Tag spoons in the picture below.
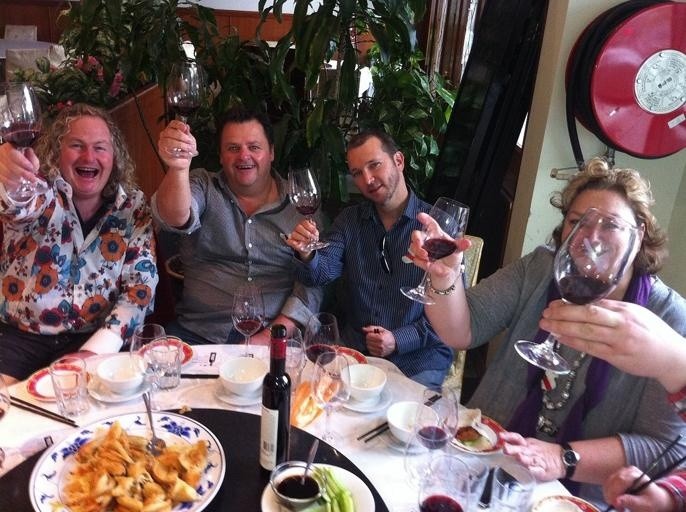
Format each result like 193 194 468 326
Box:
142 392 166 456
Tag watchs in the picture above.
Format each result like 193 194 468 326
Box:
555 439 580 481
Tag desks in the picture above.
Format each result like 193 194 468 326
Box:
0 38 56 61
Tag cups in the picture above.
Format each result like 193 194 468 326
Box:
150 334 181 391
490 464 535 512
449 459 491 511
418 457 470 511
52 355 90 419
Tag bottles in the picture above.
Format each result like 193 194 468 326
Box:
258 324 293 473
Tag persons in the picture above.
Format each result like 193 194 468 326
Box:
0 103 160 392
151 103 329 348
409 155 686 512
287 130 468 393
537 298 685 512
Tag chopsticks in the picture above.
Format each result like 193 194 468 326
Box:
7 394 79 430
605 435 686 512
358 394 442 444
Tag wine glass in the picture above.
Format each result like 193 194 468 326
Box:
165 60 204 156
130 321 165 413
400 198 471 305
313 352 349 440
233 286 265 357
305 313 341 373
415 388 458 470
0 81 47 199
280 326 308 390
515 209 644 374
288 169 330 252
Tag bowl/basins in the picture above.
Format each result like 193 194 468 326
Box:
97 355 146 396
342 364 387 396
387 401 437 442
215 358 267 395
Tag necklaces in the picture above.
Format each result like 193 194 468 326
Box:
539 348 586 416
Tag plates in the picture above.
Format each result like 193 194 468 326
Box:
532 495 603 512
317 347 367 377
140 339 191 367
213 385 263 407
28 411 225 511
29 363 92 403
375 417 449 454
260 465 377 512
86 376 154 403
267 462 323 509
443 417 506 455
340 385 394 412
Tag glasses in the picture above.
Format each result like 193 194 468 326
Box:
379 233 393 276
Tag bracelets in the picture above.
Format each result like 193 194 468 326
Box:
425 263 464 295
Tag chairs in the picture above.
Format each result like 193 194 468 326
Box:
445 235 485 398
5 49 52 82
143 228 184 345
50 45 77 70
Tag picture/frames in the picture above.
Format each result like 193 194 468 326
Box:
4 25 38 42
